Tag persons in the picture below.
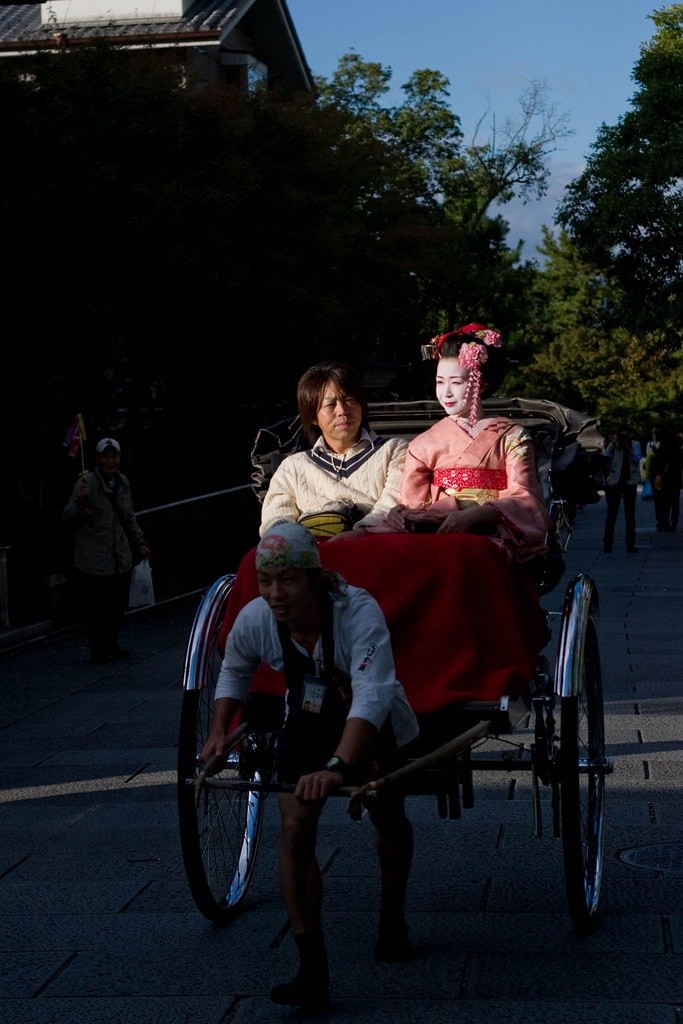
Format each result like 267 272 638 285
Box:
388 323 552 569
303 701 310 711
646 429 683 533
259 362 410 544
602 423 640 553
60 437 150 664
200 523 413 1002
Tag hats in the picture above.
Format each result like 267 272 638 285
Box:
96 438 121 452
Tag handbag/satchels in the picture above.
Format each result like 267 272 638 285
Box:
641 480 653 499
128 558 155 608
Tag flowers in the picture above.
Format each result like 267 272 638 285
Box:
457 342 489 426
475 330 502 348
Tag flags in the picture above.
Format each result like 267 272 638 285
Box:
64 415 86 456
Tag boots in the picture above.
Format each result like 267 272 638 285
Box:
270 932 330 1005
374 877 406 962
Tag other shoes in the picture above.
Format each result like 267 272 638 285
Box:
105 643 141 664
627 546 639 553
74 641 102 665
604 547 612 553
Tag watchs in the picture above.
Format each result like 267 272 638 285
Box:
326 757 349 781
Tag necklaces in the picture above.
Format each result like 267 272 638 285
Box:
330 451 348 480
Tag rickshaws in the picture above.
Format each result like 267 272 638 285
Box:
176 397 613 922
